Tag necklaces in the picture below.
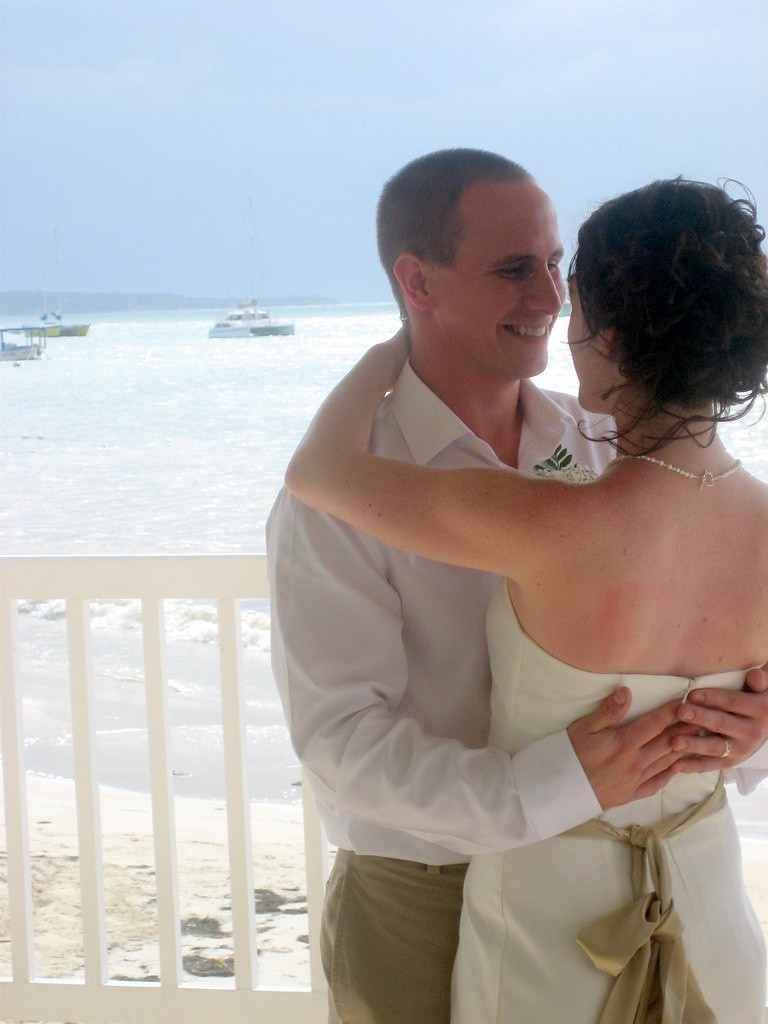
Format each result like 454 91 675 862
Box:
607 455 742 491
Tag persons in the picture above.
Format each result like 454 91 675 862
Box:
260 147 767 1024
277 176 766 1023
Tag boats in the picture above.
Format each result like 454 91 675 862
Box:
0 328 44 361
209 302 294 337
22 322 89 336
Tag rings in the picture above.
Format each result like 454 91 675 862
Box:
720 737 732 758
